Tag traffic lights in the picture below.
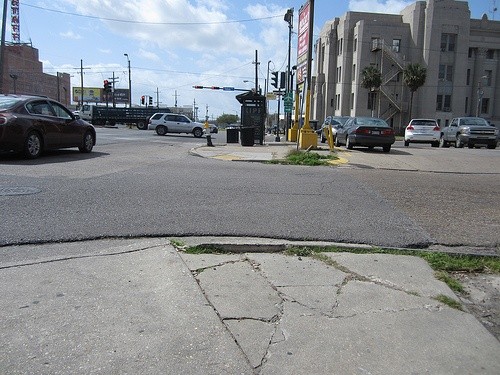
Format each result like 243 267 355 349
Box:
271 72 278 88
149 96 153 105
103 80 109 93
141 96 145 104
108 82 112 92
210 86 220 90
195 85 204 90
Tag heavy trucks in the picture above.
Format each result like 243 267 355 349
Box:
68 104 172 130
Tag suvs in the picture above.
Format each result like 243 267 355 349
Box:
147 113 205 138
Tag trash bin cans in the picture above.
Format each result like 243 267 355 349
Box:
226 129 239 143
240 127 254 147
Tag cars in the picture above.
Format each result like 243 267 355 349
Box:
267 125 285 135
0 93 97 160
334 116 395 153
319 116 353 145
402 119 441 148
442 117 497 149
208 124 218 135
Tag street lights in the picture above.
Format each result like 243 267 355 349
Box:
9 73 18 93
124 53 132 108
476 76 489 118
